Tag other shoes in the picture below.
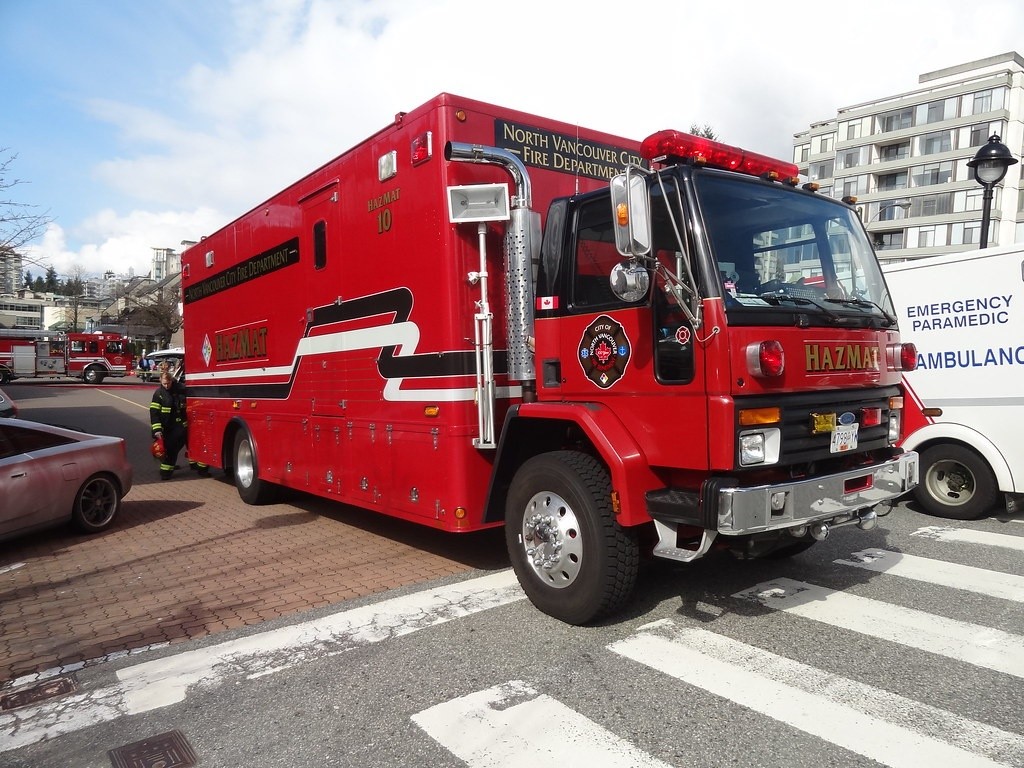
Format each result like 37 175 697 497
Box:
195 466 211 478
162 473 171 480
175 465 181 470
190 464 198 469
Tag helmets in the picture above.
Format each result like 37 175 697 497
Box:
151 436 166 459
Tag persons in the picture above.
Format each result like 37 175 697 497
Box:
149 371 214 482
174 361 182 373
156 356 171 381
138 351 153 383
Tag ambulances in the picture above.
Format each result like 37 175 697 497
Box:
797 241 1024 521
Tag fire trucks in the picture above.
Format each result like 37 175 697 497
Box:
181 91 917 631
0 328 134 386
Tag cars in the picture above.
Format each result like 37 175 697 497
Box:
0 388 18 419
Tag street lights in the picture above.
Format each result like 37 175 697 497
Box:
966 130 1019 249
850 200 911 297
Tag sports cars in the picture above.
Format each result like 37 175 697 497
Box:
0 417 134 548
